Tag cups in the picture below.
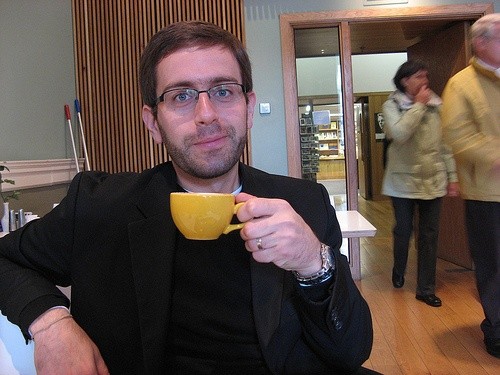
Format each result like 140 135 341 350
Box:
170 192 252 241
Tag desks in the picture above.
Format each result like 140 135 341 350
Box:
317 159 346 180
335 210 377 281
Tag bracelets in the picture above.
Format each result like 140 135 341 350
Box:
33 313 74 340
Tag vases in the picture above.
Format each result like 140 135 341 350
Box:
0 203 10 233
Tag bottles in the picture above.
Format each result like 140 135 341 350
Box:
18 209 25 228
10 210 16 231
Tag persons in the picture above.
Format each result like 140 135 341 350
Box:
440 11 500 357
0 16 391 375
383 58 459 307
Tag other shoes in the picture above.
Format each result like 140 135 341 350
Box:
415 293 442 307
392 268 404 288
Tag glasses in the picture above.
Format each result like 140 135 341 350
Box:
154 82 249 112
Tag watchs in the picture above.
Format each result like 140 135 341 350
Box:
293 241 335 285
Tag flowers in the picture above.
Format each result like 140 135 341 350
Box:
0 161 21 203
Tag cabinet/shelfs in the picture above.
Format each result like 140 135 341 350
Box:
300 114 319 182
318 120 342 155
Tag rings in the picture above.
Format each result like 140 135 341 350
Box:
255 237 264 251
